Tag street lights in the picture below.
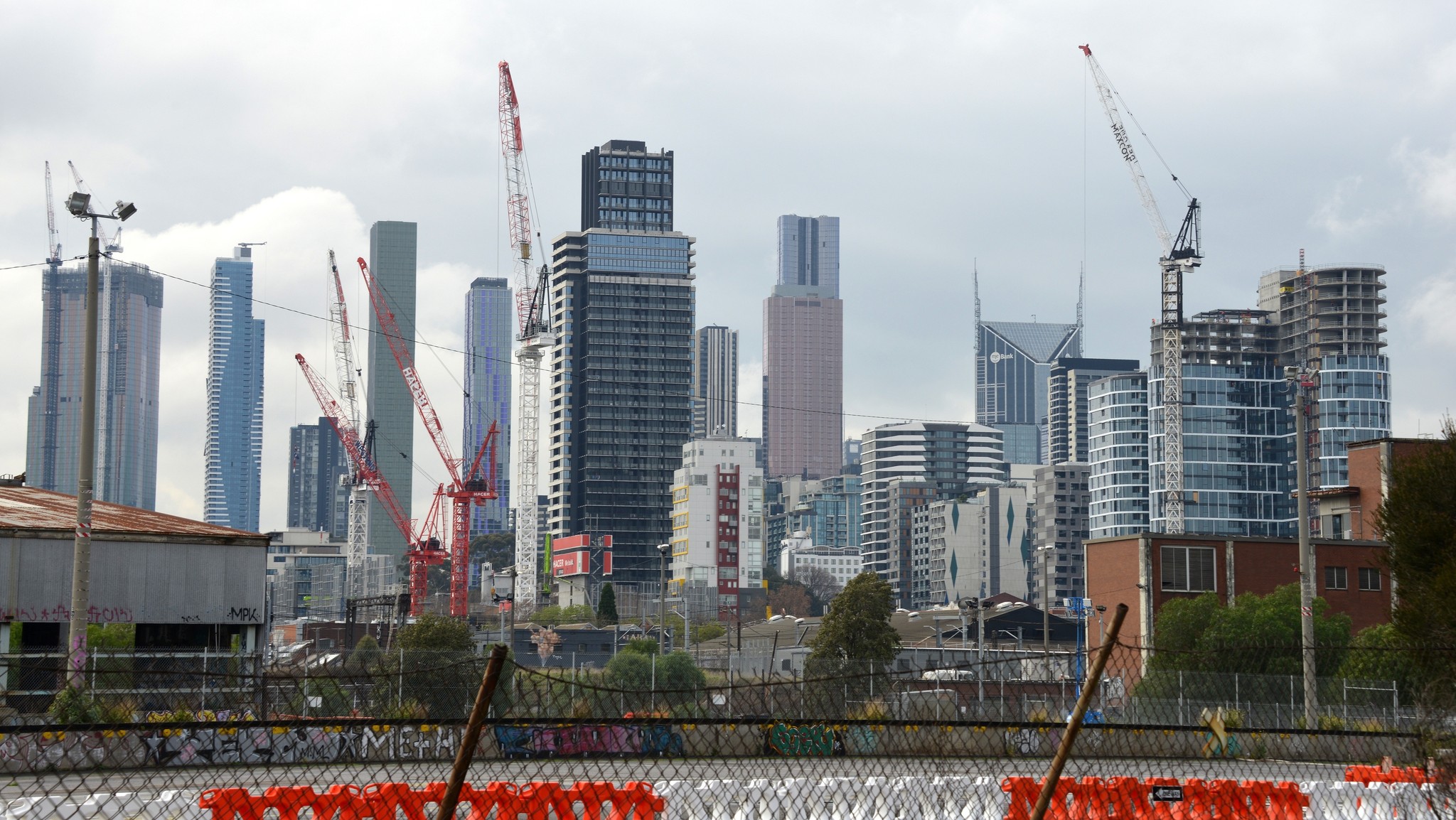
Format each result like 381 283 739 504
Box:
61 188 138 695
658 544 670 654
1038 545 1054 679
1096 605 1106 712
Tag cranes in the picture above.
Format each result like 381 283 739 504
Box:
358 257 501 616
295 352 449 618
67 159 124 501
40 160 64 491
494 57 558 622
326 249 382 618
1076 43 1207 534
